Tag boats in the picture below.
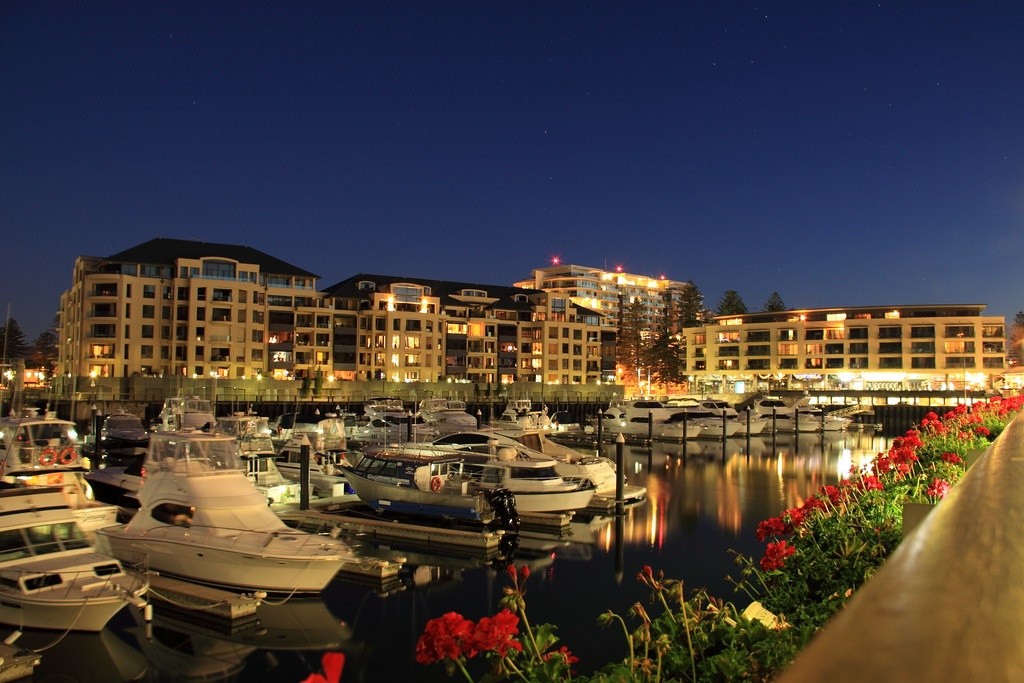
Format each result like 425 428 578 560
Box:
650 440 702 458
0 628 44 683
566 511 619 546
359 536 508 589
0 397 855 633
823 429 851 442
734 435 766 453
514 526 570 576
136 562 353 683
0 620 150 683
768 432 819 446
698 438 740 457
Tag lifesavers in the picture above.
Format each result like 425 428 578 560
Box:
39 449 57 466
59 447 78 464
431 477 441 492
